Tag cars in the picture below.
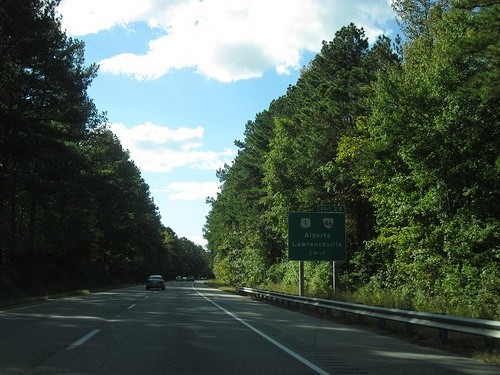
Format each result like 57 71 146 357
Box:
175 275 195 282
146 275 165 290
